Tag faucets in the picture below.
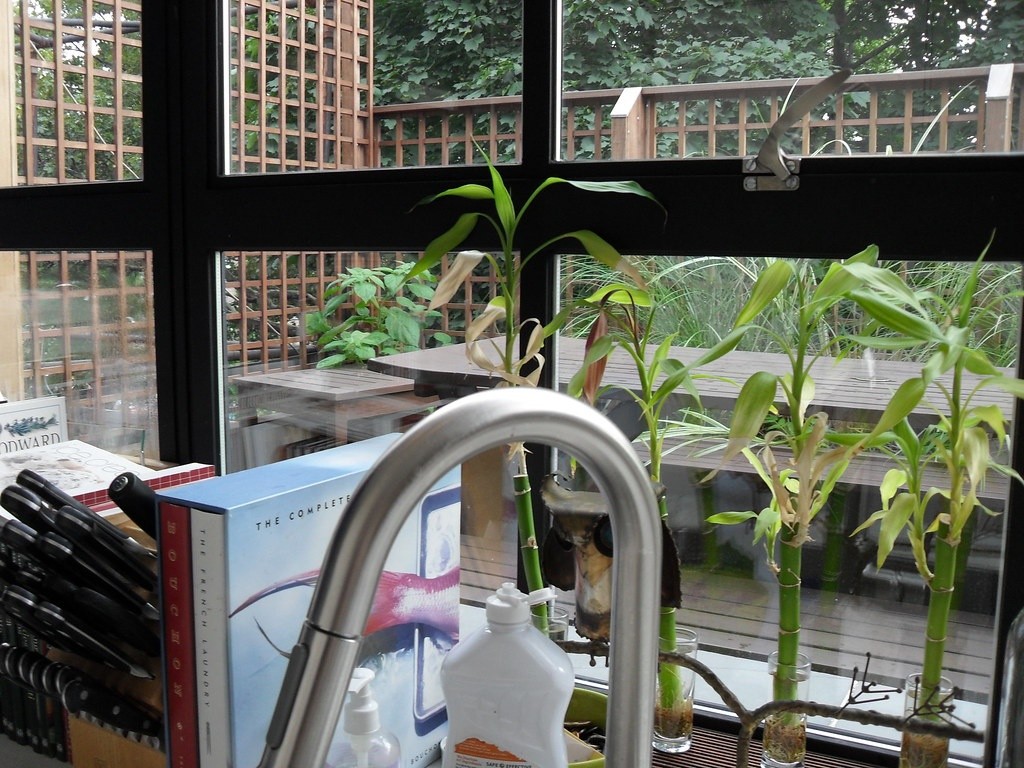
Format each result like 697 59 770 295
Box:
258 386 661 768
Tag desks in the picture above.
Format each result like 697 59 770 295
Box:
370 332 1018 565
230 369 438 448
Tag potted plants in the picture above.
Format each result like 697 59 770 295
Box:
714 243 930 768
549 252 739 753
840 228 1019 768
396 135 659 639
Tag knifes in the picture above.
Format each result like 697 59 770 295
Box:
0 469 159 750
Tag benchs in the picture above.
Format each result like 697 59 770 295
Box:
630 438 1010 557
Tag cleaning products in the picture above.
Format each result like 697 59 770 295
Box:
324 667 401 767
440 581 576 768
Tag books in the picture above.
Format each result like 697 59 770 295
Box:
0 395 215 526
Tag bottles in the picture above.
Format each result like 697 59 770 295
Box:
440 583 575 768
326 667 402 768
